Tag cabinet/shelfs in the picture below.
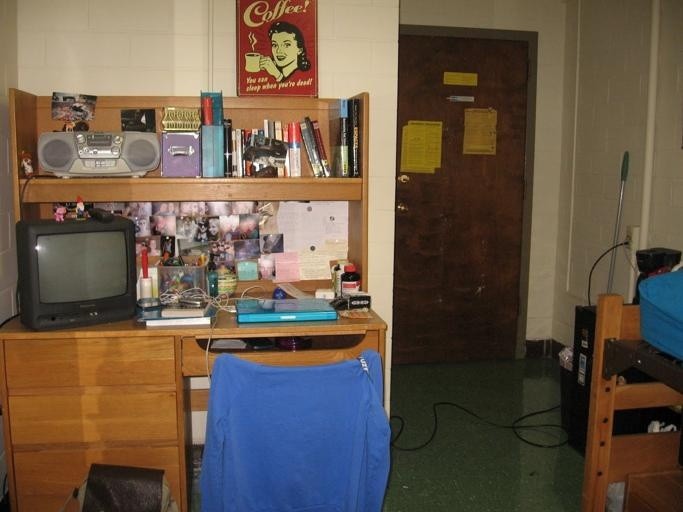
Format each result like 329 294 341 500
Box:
181 292 385 512
9 88 370 211
3 311 186 509
581 294 682 510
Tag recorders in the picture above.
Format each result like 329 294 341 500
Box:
38 130 161 180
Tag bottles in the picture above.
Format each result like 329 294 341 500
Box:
340 265 360 297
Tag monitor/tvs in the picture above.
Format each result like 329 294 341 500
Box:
15 214 138 331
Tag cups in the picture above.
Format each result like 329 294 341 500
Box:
244 53 264 73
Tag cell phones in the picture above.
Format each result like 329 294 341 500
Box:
179 297 205 308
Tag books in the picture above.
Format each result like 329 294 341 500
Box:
223 98 359 180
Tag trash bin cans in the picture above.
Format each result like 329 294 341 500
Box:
559 347 575 432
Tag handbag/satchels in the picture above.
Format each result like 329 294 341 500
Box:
75 462 180 511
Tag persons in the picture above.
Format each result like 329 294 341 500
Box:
128 201 282 267
